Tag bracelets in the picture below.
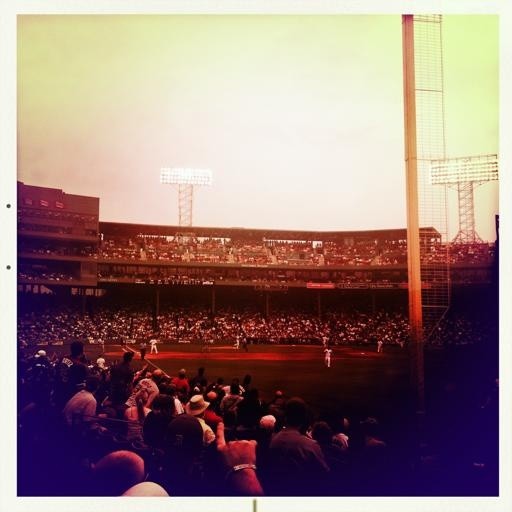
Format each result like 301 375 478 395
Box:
225 464 256 480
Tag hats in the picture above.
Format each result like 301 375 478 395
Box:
260 415 276 428
186 394 209 416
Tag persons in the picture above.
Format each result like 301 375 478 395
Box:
98 337 105 352
245 415 276 448
265 311 322 345
383 340 409 350
95 352 140 496
241 335 248 352
235 311 265 335
234 334 240 350
19 271 77 280
218 374 269 421
217 422 264 497
86 305 155 338
215 311 234 344
17 309 86 345
322 311 377 345
378 310 409 339
150 337 158 354
324 346 332 367
140 340 147 361
100 230 276 283
156 306 215 345
121 338 123 347
23 243 100 258
269 397 330 497
311 411 386 467
424 311 481 345
17 339 98 497
248 335 265 344
377 339 383 352
273 240 496 282
141 364 226 496
